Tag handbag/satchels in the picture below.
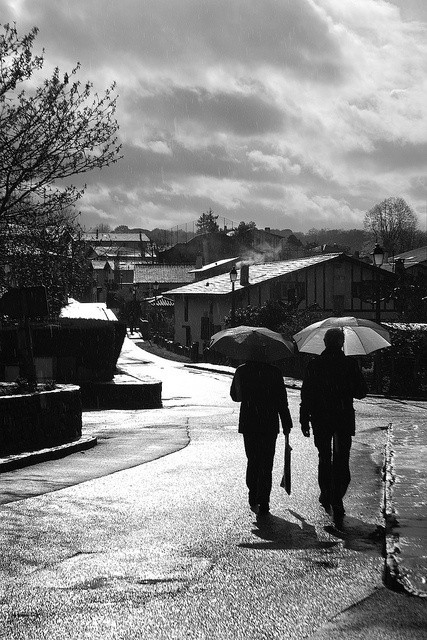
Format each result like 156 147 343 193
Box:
280 434 293 495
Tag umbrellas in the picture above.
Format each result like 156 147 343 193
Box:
293 316 392 356
209 326 293 363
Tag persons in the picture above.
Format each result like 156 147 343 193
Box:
230 349 293 515
119 312 127 336
128 312 135 335
300 329 367 520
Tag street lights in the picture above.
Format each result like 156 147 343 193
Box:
368 243 383 323
230 266 236 327
119 273 123 290
133 283 138 331
153 281 159 343
